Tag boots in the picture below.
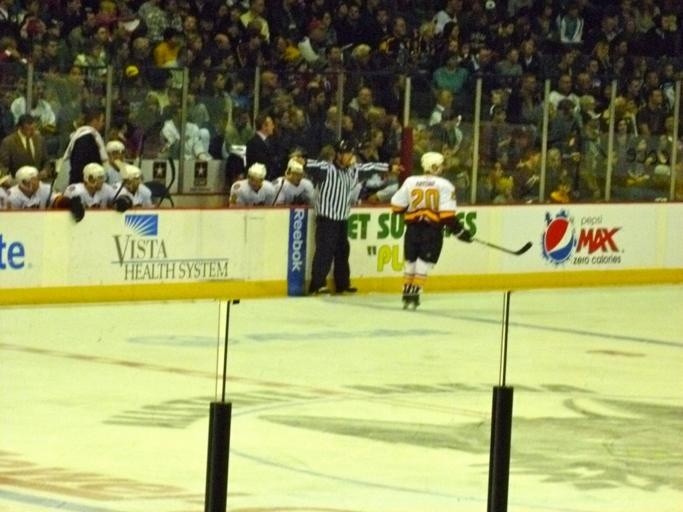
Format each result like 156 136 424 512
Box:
402 282 420 305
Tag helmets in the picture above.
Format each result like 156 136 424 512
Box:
120 164 142 181
248 163 267 181
107 140 126 162
285 160 304 186
15 165 39 191
421 152 445 175
332 139 358 154
83 163 107 189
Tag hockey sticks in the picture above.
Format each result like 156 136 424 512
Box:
155 156 175 208
109 160 130 210
473 237 532 256
45 160 57 210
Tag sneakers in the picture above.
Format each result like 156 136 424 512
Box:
312 286 331 293
335 287 357 293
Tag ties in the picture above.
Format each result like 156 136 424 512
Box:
25 137 33 158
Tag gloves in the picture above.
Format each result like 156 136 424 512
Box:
451 223 473 243
68 195 85 222
114 194 132 213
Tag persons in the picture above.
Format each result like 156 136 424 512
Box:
393 150 474 310
0 0 682 224
292 136 407 298
